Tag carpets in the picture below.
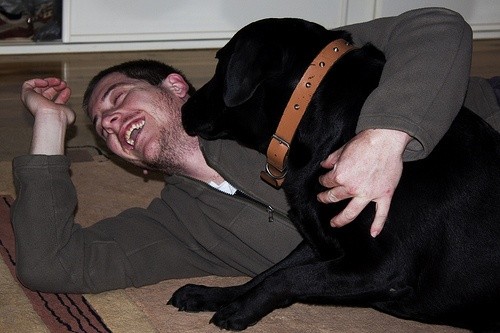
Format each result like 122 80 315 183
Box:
0 146 481 333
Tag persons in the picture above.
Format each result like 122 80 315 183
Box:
11 6 500 293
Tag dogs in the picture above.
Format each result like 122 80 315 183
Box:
165 17 500 333
